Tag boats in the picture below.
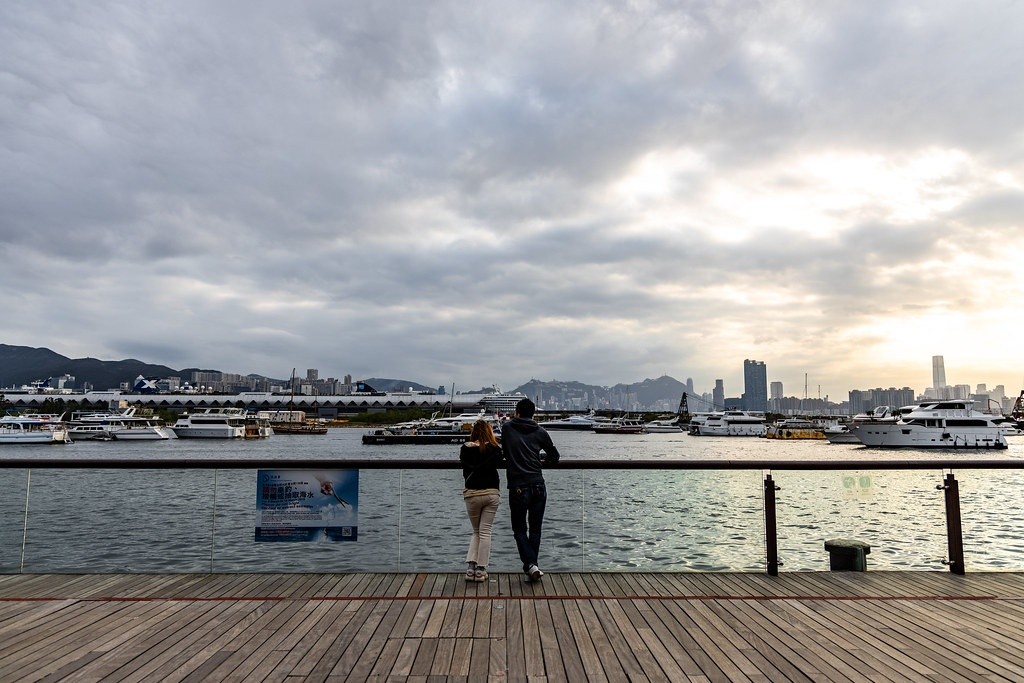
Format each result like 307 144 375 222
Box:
814 399 1024 450
536 408 768 436
383 382 511 434
0 368 328 444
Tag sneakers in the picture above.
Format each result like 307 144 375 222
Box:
475 569 489 581
465 569 475 582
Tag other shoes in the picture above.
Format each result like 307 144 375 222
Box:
524 574 531 582
529 564 544 580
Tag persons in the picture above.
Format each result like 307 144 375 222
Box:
502 398 560 582
459 419 503 582
